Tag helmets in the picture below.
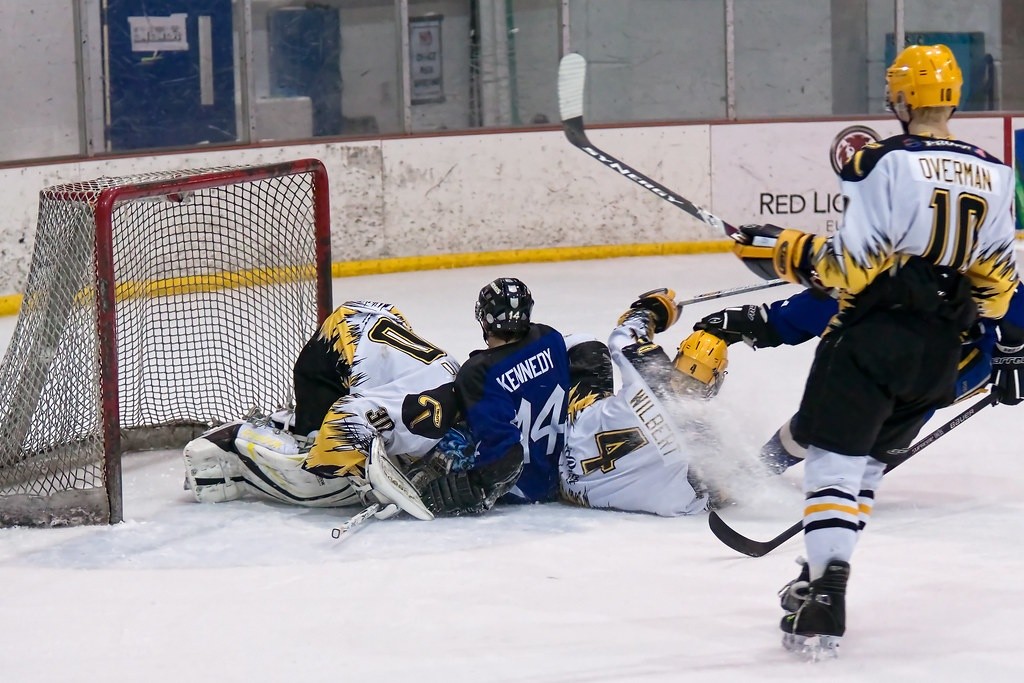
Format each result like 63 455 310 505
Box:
885 45 963 112
474 278 535 333
674 330 729 386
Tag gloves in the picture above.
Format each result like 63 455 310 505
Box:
734 224 811 283
691 302 769 350
422 471 483 518
631 287 684 332
990 326 1024 406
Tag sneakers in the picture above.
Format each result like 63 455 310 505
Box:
778 562 810 611
780 560 850 637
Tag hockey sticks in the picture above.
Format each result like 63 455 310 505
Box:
705 391 995 559
326 497 387 542
555 50 747 244
674 280 794 308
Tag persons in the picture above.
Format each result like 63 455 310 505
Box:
734 43 1020 663
183 279 1024 518
